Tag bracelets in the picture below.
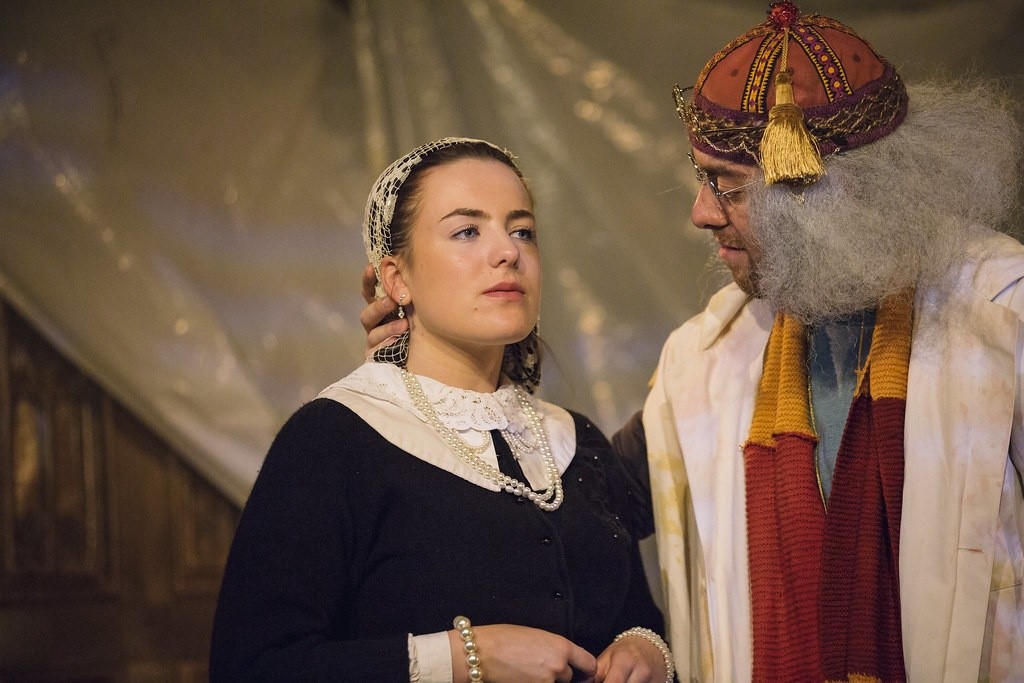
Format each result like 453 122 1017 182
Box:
453 615 483 682
613 626 676 683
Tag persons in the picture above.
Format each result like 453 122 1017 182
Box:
206 137 681 683
358 0 1024 683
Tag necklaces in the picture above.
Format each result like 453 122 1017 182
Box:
401 364 565 512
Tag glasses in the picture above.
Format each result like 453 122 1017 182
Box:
686 151 771 213
671 83 770 144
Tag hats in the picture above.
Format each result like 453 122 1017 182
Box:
687 0 910 186
